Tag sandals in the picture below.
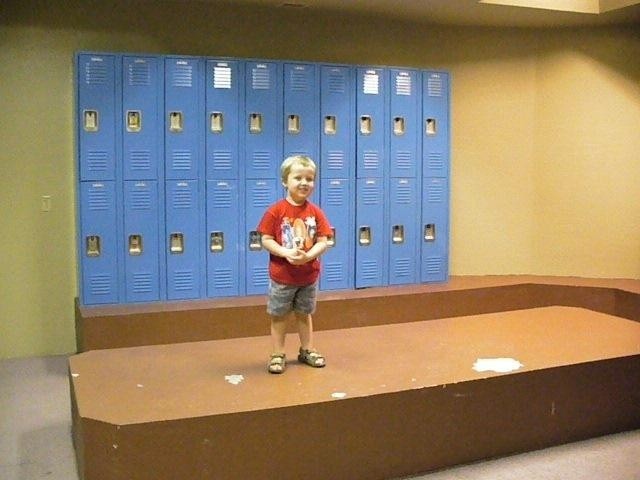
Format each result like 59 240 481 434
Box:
299 347 325 367
268 352 285 373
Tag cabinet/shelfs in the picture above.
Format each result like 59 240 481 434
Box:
75 51 450 307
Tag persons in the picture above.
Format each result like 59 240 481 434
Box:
257 154 335 374
